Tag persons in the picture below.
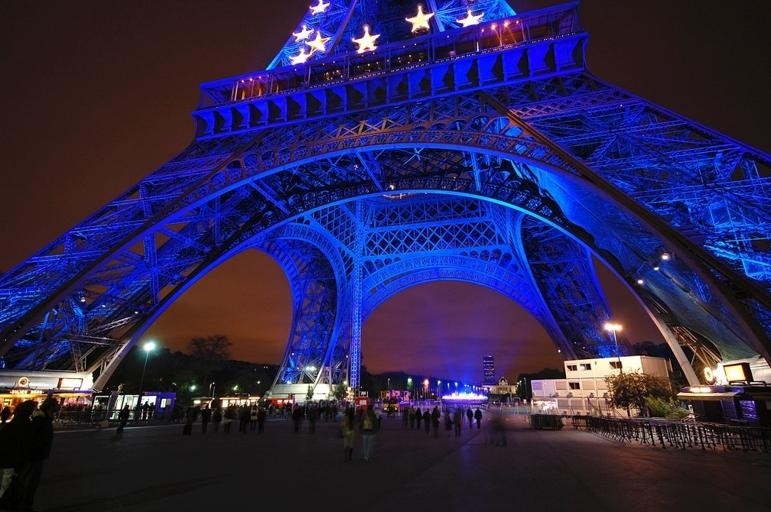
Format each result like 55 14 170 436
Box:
116 405 130 433
172 402 379 462
133 403 155 424
1 401 38 512
31 395 59 460
403 403 483 438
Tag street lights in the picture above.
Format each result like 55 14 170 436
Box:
515 376 529 401
385 377 473 400
207 382 216 396
603 320 635 419
134 341 156 410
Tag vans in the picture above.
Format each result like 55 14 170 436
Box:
383 396 399 412
